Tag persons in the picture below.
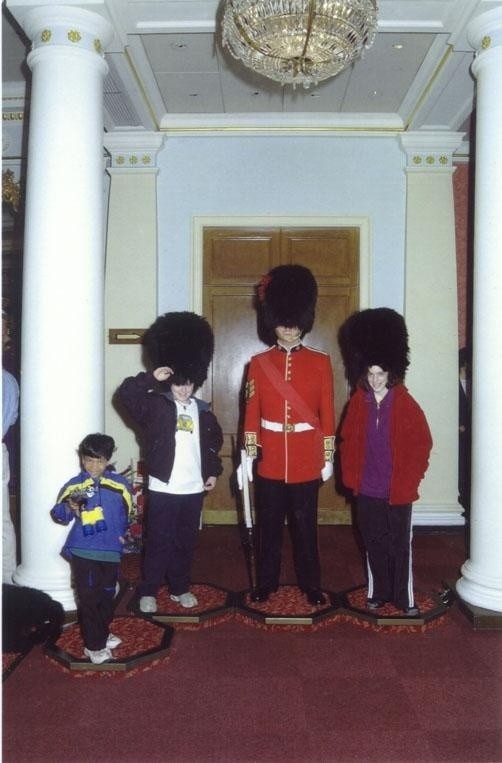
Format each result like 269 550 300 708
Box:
240 266 334 607
2 307 20 584
112 311 225 613
337 308 433 613
50 432 133 664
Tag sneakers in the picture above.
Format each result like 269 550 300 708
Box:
140 595 159 614
84 646 113 665
170 591 199 608
105 633 123 650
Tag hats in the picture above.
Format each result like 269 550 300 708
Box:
255 264 318 347
143 312 214 391
338 308 409 388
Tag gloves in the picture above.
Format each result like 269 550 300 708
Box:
236 458 254 488
321 461 335 481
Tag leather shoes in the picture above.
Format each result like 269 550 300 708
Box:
251 584 279 603
396 600 420 618
366 597 389 610
303 587 327 605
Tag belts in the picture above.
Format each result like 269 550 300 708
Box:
261 418 315 433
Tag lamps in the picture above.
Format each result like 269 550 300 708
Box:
216 0 379 93
